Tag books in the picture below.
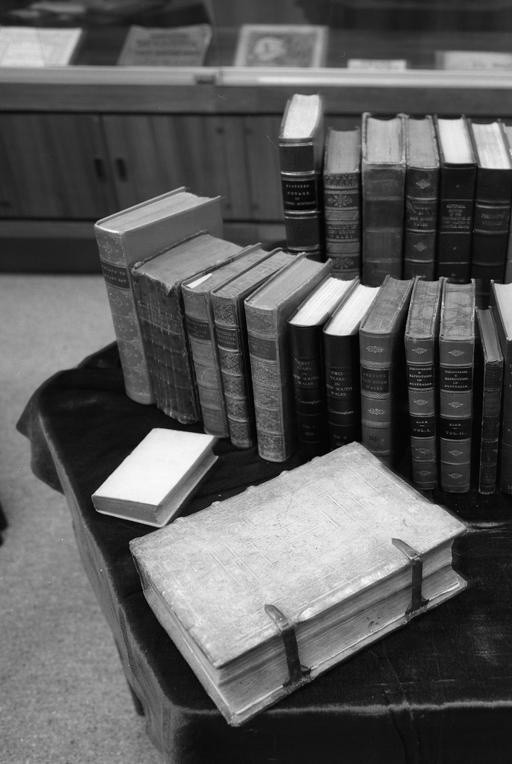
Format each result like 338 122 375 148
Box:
275 93 512 308
347 58 406 74
131 230 511 540
128 440 471 728
1 27 84 66
118 25 212 65
95 185 221 404
92 428 219 528
434 50 512 73
234 23 329 68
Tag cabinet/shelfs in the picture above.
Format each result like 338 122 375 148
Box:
1 68 510 277
17 340 510 699
